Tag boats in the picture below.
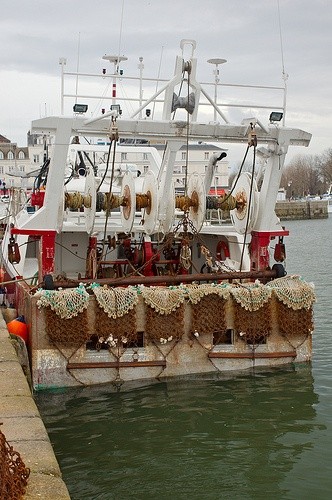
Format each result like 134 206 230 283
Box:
2 35 316 386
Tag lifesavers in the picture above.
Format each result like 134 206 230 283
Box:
216 241 229 261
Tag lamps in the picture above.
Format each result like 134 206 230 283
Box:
269 112 283 121
73 103 88 112
110 105 121 112
